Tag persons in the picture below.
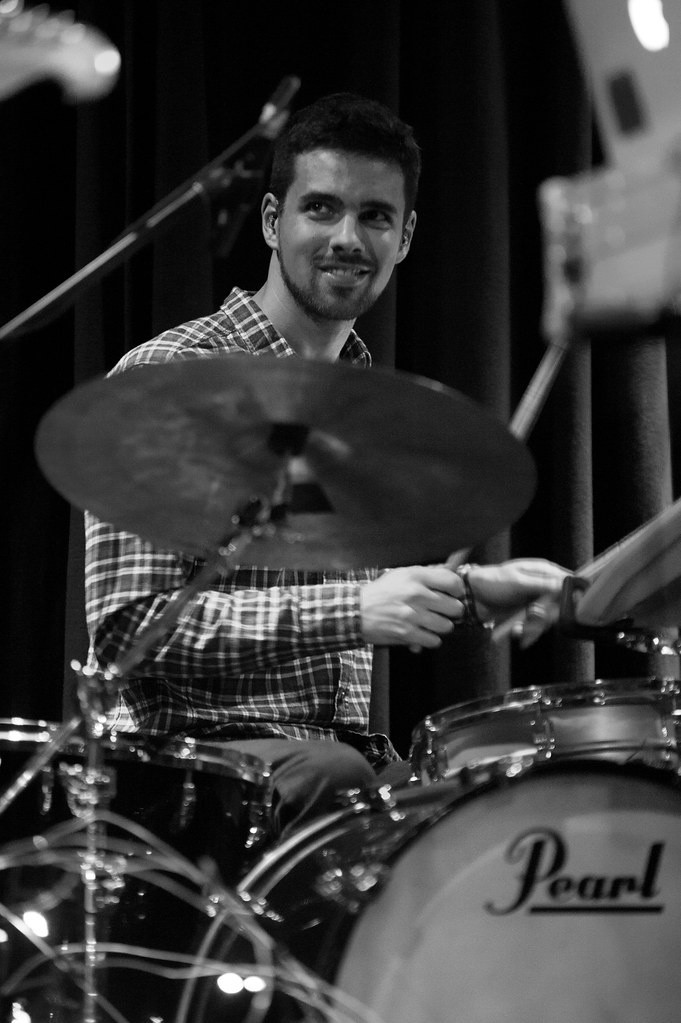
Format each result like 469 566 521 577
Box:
84 94 577 848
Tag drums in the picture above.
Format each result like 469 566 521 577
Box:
0 719 281 1022
179 761 681 1023
412 668 680 773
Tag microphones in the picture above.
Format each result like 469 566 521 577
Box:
235 75 299 180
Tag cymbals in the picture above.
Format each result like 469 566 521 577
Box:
30 351 541 582
